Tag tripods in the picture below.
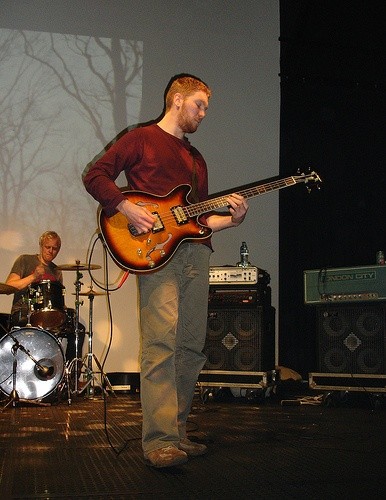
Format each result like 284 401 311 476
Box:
0 325 52 412
59 267 120 403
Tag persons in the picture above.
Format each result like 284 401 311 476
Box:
83 77 250 468
6 231 85 385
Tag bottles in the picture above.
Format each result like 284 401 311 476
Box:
240 242 249 268
376 249 385 266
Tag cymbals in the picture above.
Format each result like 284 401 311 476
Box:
53 261 103 272
0 283 20 295
72 289 111 297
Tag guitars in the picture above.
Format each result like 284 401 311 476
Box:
97 164 324 275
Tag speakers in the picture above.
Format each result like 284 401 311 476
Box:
197 303 278 390
310 303 386 394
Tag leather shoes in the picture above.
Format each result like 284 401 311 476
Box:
179 439 208 456
147 444 188 469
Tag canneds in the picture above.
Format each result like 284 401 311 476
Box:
376 251 384 265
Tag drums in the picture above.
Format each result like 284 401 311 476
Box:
47 307 76 337
0 327 66 401
22 279 67 329
